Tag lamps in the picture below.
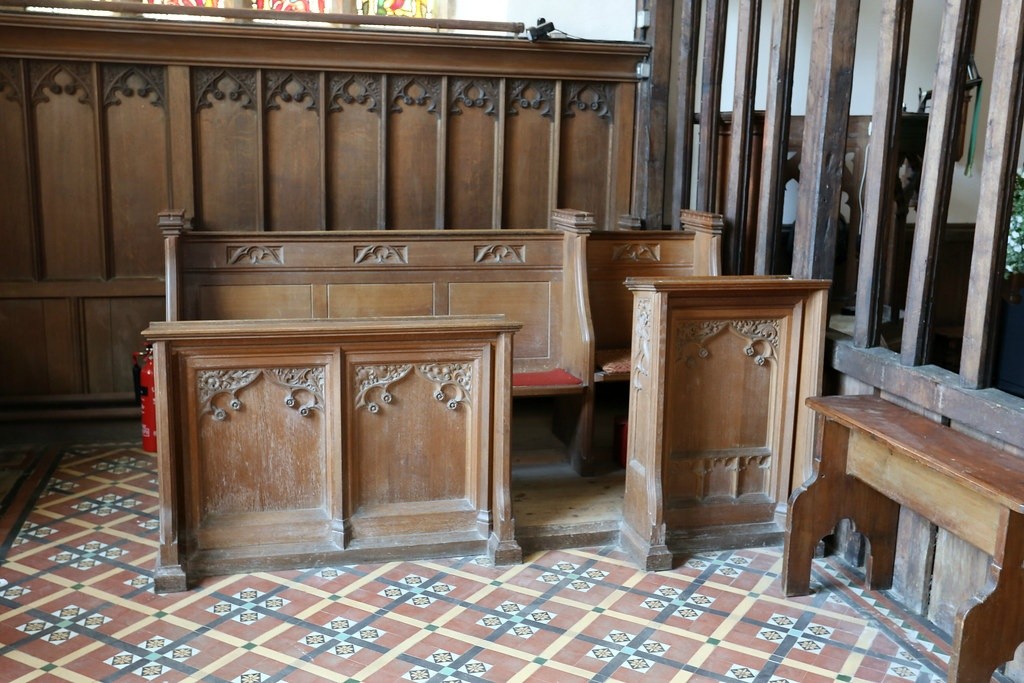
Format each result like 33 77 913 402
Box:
526 18 555 43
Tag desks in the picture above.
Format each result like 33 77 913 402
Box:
782 393 1024 683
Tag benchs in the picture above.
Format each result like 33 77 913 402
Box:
157 208 724 478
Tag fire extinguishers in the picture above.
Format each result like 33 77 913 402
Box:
132 342 157 452
